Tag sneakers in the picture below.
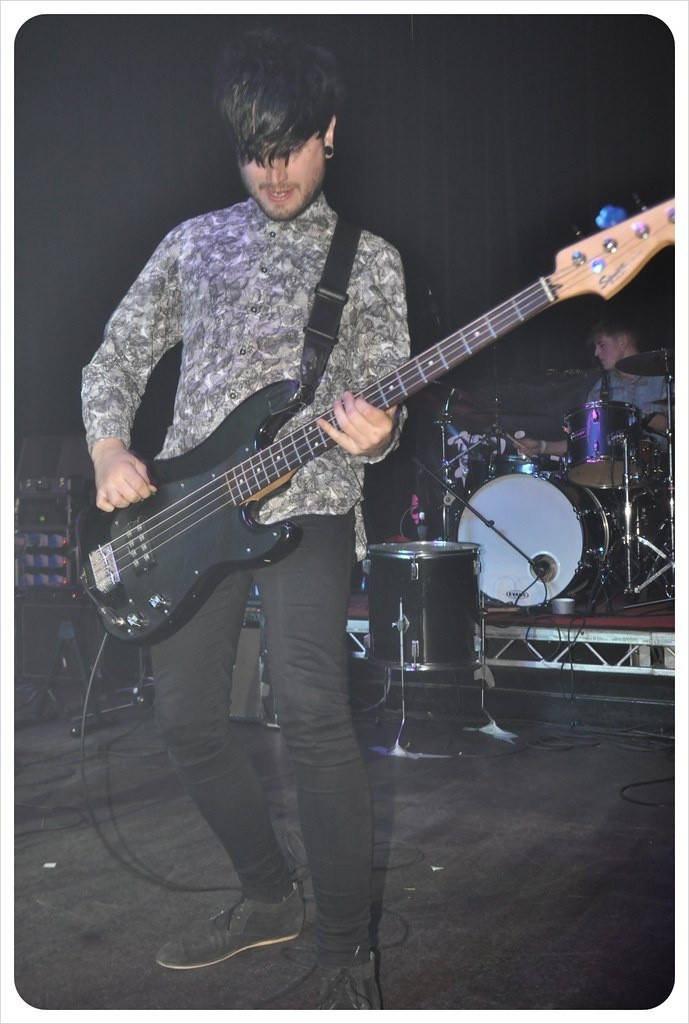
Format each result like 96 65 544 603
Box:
256 946 381 1009
155 881 305 970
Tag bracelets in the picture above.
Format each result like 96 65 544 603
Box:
540 439 546 456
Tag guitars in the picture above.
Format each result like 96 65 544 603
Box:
75 190 675 641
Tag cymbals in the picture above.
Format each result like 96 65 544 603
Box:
615 348 675 376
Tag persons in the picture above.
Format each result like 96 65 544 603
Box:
81 42 410 1010
513 312 675 457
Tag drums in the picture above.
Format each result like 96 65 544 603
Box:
365 400 645 672
